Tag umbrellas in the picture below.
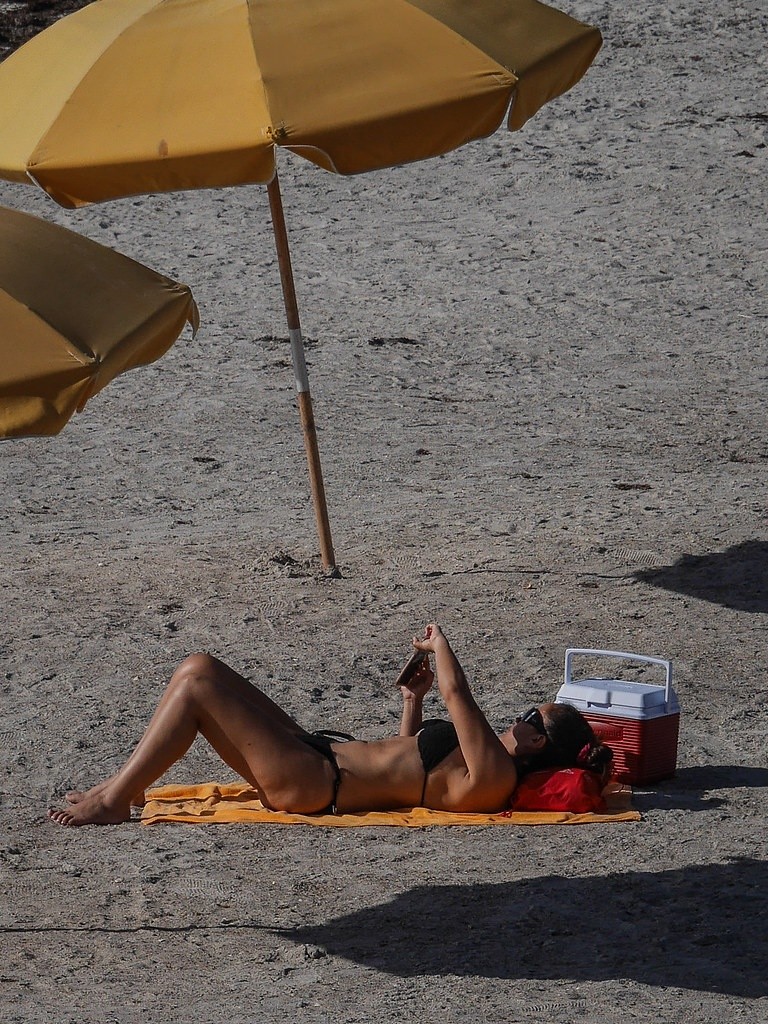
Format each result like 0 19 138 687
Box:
0 0 602 570
0 207 200 442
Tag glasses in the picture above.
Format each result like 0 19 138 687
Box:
520 707 547 736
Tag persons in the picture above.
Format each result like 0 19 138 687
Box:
46 623 614 826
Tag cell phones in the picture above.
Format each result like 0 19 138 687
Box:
394 637 432 687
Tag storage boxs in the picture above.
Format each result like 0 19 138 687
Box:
554 645 682 788
